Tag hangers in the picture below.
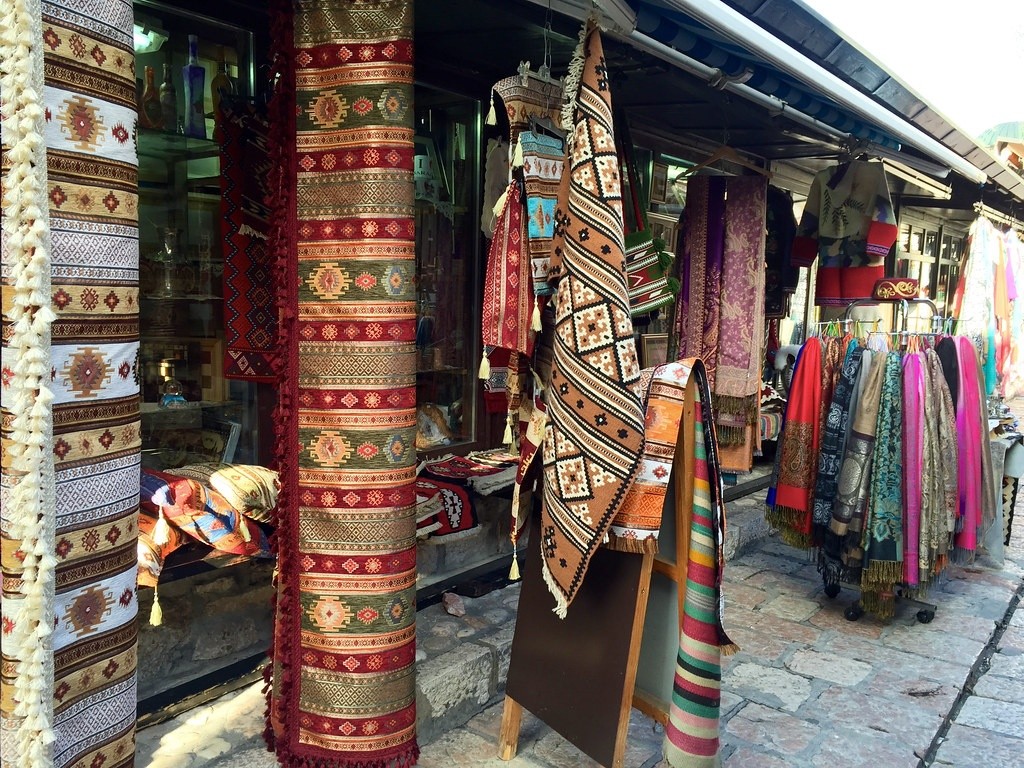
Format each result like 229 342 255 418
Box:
518 22 564 90
216 64 272 113
674 129 774 185
527 82 568 153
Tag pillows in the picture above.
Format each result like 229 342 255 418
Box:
760 378 787 413
163 462 280 523
140 466 274 558
137 513 196 626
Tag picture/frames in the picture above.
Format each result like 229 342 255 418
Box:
640 334 669 368
148 413 242 464
415 129 451 201
650 162 668 204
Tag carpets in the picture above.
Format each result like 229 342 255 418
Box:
418 8 769 768
216 98 277 384
0 2 141 768
261 0 420 768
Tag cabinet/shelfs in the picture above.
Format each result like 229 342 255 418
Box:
138 127 242 412
414 203 470 463
